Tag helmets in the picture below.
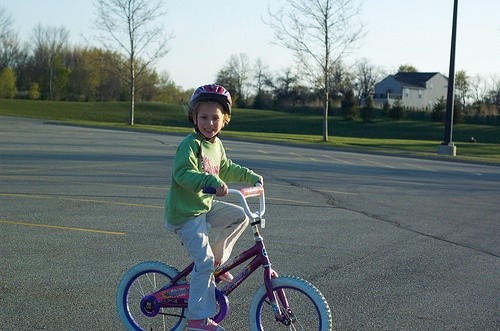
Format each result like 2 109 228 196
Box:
188 84 233 126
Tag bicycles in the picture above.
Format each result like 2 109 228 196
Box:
115 182 333 331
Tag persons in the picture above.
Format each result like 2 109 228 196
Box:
164 84 263 331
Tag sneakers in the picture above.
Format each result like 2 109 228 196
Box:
213 261 233 282
187 318 226 331
